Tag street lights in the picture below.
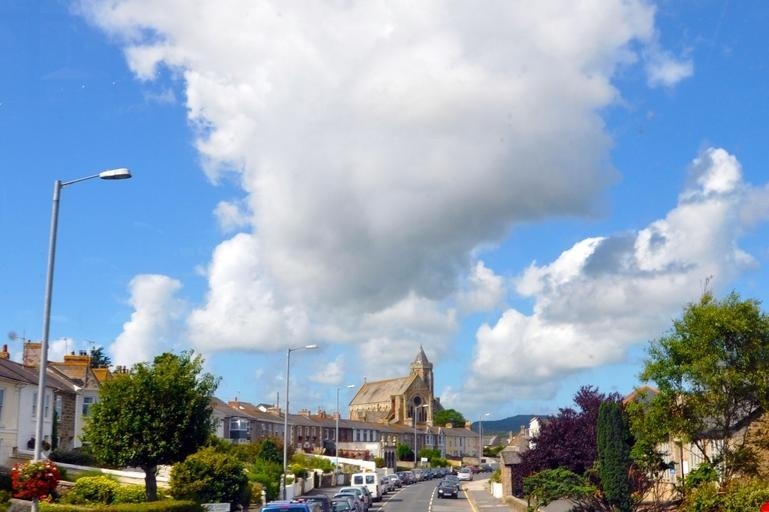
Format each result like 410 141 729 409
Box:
673 392 687 500
283 343 319 501
414 404 429 469
335 385 355 473
33 167 131 462
478 413 490 462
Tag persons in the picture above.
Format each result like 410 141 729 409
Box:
40 434 51 458
28 437 35 450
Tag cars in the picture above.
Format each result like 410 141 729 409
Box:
260 463 492 512
